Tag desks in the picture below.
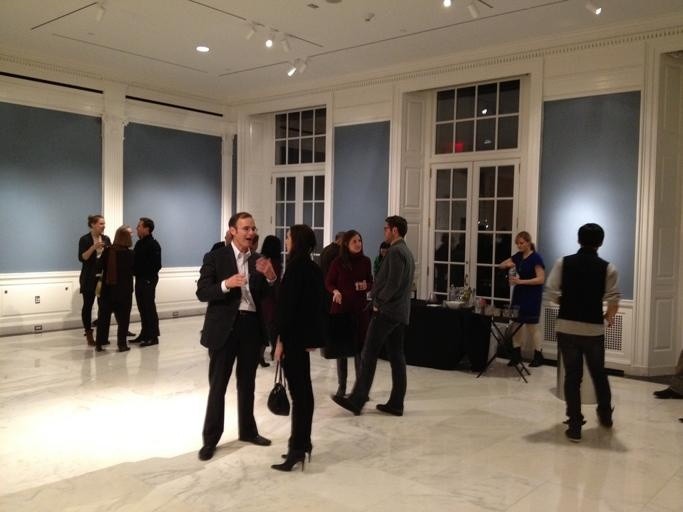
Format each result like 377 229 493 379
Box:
472 308 540 383
368 296 493 375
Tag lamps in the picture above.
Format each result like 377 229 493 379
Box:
286 58 307 77
245 24 291 54
443 1 481 21
95 4 107 23
585 1 603 16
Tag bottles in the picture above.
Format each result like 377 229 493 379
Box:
409 282 417 299
508 266 517 286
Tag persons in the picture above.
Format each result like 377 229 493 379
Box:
652 350 681 402
74 215 136 347
497 231 545 367
212 228 392 397
429 225 509 302
195 213 271 460
544 223 620 443
127 217 163 347
331 216 415 416
95 224 135 352
268 224 319 471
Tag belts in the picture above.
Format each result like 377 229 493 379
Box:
237 310 248 316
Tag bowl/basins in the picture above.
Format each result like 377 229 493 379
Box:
445 300 464 310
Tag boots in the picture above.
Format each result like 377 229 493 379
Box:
529 348 545 367
85 329 95 345
507 346 522 366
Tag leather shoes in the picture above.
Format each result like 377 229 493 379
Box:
200 446 214 459
96 330 158 351
260 357 270 366
239 435 271 445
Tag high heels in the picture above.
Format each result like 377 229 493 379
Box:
272 443 313 471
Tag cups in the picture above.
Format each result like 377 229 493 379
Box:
474 298 521 319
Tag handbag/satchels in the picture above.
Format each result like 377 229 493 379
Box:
268 383 289 415
95 281 102 297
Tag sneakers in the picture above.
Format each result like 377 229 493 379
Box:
565 429 582 442
334 390 369 412
654 388 683 399
377 404 403 416
598 416 613 427
